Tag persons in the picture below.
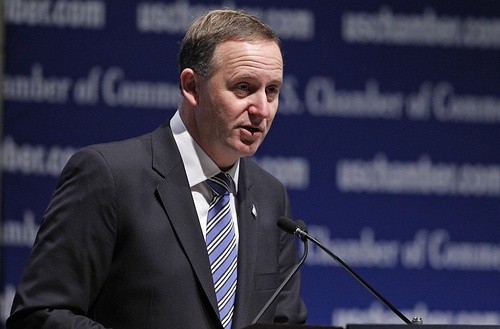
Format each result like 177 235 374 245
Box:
2 7 308 329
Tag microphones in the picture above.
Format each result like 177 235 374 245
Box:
276 216 414 325
251 220 309 325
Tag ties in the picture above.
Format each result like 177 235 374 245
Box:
207 172 239 329
